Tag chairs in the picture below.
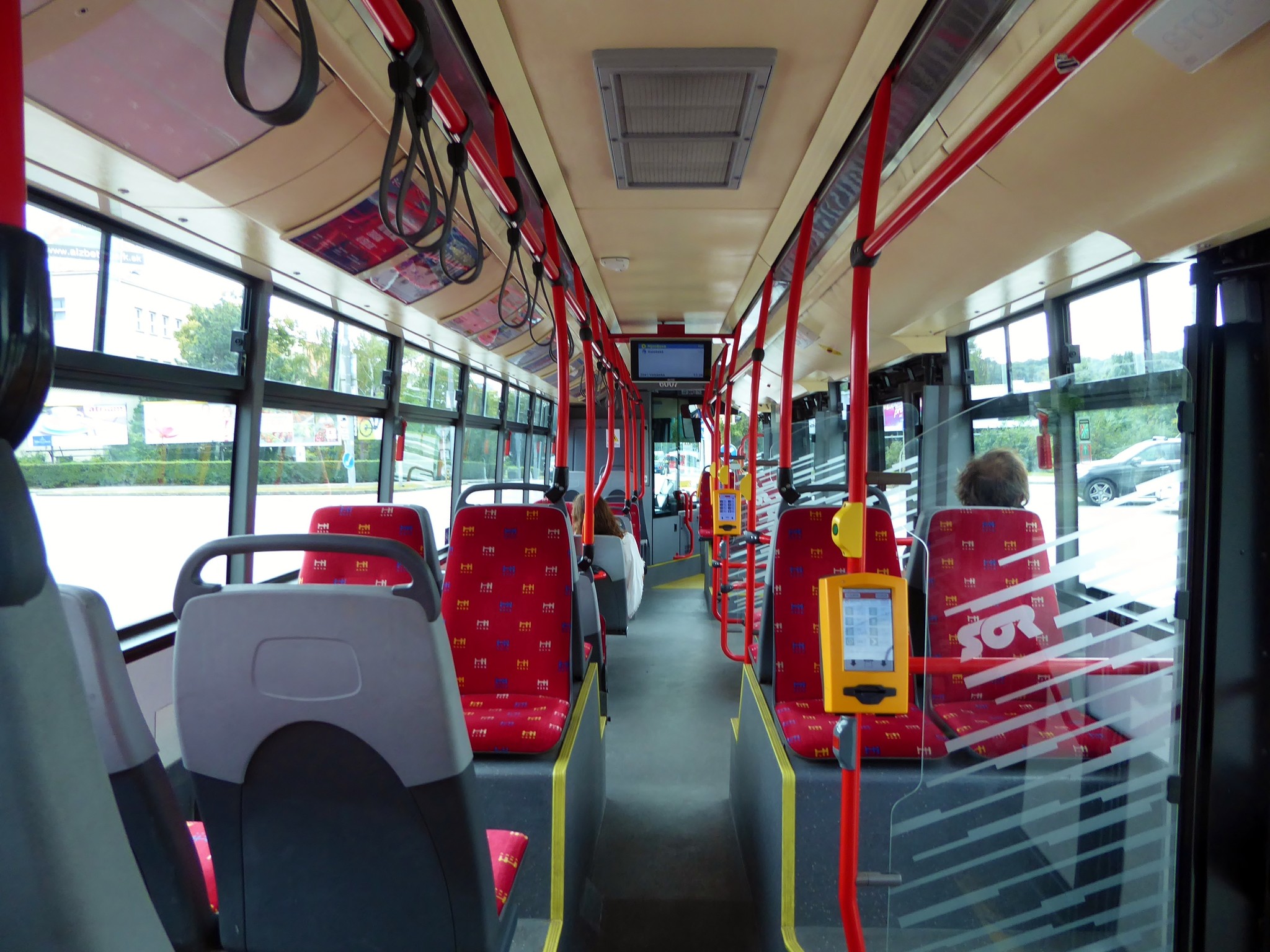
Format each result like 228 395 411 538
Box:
0 465 1131 952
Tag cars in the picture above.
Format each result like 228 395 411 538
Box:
655 462 664 475
667 456 678 468
1076 436 1182 506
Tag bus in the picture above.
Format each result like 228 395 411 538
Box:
680 454 685 474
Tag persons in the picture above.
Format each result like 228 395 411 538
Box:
568 494 647 620
959 449 1033 507
